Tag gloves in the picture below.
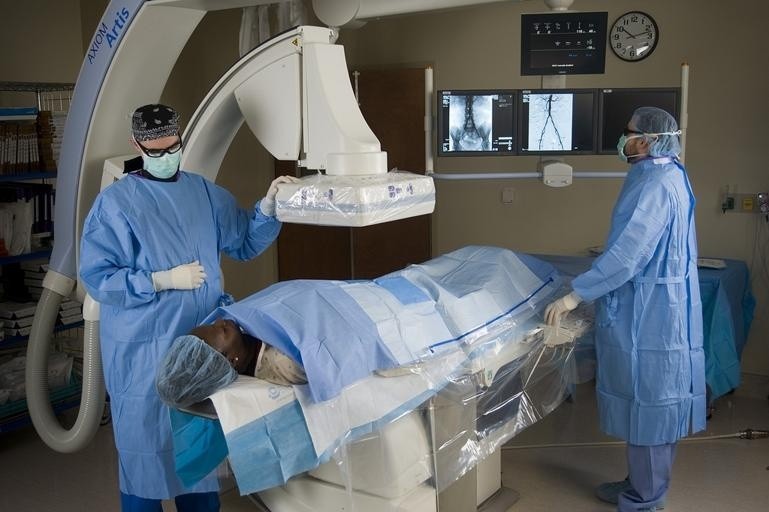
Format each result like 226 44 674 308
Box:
543 289 584 327
150 259 208 295
259 174 301 219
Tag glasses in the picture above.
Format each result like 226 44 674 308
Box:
132 133 183 159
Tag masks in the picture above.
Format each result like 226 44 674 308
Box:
616 133 648 163
133 135 183 179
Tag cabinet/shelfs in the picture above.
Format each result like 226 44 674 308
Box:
0 81 112 427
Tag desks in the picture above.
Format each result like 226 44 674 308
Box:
529 254 755 419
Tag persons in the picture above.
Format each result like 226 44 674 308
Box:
77 104 300 512
158 318 305 411
542 105 696 511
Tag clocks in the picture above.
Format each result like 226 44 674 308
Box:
609 11 659 63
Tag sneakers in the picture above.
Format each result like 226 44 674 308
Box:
595 480 630 505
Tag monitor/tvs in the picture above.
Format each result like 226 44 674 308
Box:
598 87 681 155
436 90 520 158
519 10 608 78
517 87 598 156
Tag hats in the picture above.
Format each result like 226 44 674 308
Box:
131 103 182 140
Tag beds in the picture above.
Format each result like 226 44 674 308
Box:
177 284 595 512
